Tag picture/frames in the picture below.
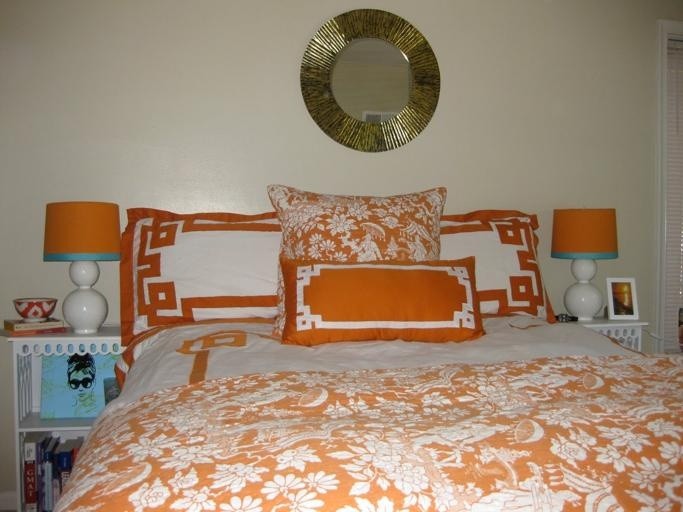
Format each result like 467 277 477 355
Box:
606 278 638 321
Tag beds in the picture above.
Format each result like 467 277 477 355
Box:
55 182 681 512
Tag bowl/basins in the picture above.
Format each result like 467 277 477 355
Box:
12 296 60 322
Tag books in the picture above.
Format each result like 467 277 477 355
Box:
1 319 68 338
20 433 82 512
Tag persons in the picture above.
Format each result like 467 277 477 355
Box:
66 353 98 407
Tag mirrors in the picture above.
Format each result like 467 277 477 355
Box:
299 9 441 152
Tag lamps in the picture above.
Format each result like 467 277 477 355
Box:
43 203 122 337
550 207 618 321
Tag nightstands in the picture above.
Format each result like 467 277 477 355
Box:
1 319 121 512
559 317 648 352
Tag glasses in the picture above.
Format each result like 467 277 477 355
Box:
69 377 94 389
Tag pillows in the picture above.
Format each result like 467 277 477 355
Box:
278 255 483 346
119 208 557 346
268 185 444 345
